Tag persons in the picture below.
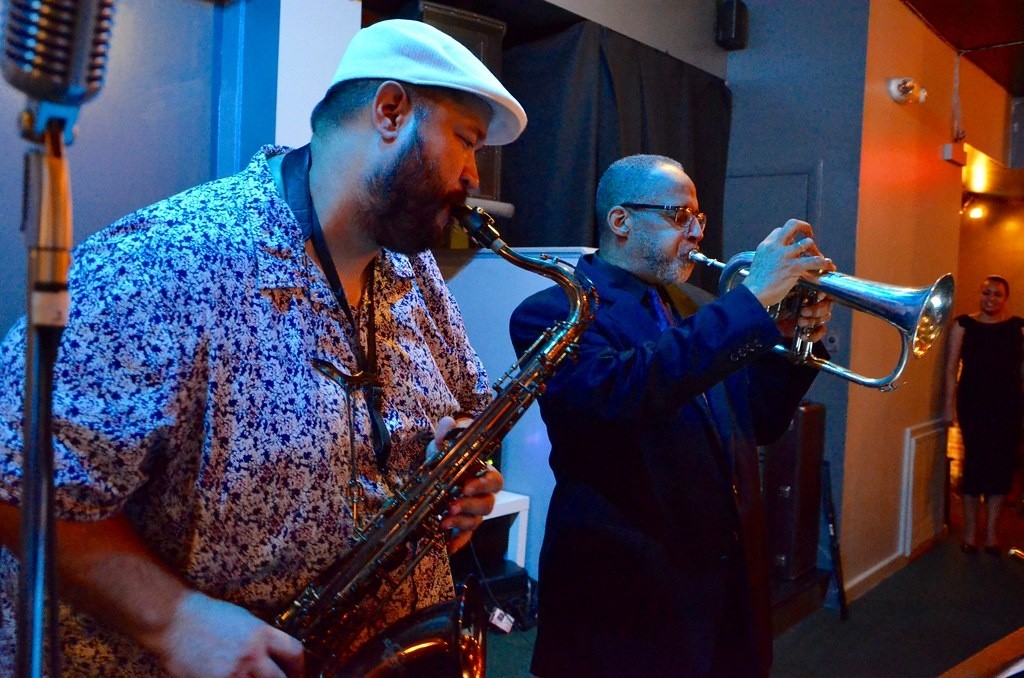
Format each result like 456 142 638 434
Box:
942 275 1024 558
509 155 836 678
0 18 527 678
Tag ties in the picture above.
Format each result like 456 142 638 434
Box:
645 284 715 408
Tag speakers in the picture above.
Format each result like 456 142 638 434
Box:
716 0 749 51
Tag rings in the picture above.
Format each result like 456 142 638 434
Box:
796 241 806 253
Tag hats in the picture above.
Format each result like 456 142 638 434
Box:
329 19 528 147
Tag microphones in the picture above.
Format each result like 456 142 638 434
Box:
0 0 117 144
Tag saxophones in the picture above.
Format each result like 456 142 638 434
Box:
262 197 616 678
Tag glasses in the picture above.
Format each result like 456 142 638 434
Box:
618 202 708 234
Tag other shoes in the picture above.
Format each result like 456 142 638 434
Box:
958 540 977 555
981 543 1004 559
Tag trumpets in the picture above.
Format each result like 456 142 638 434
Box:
685 249 957 396
